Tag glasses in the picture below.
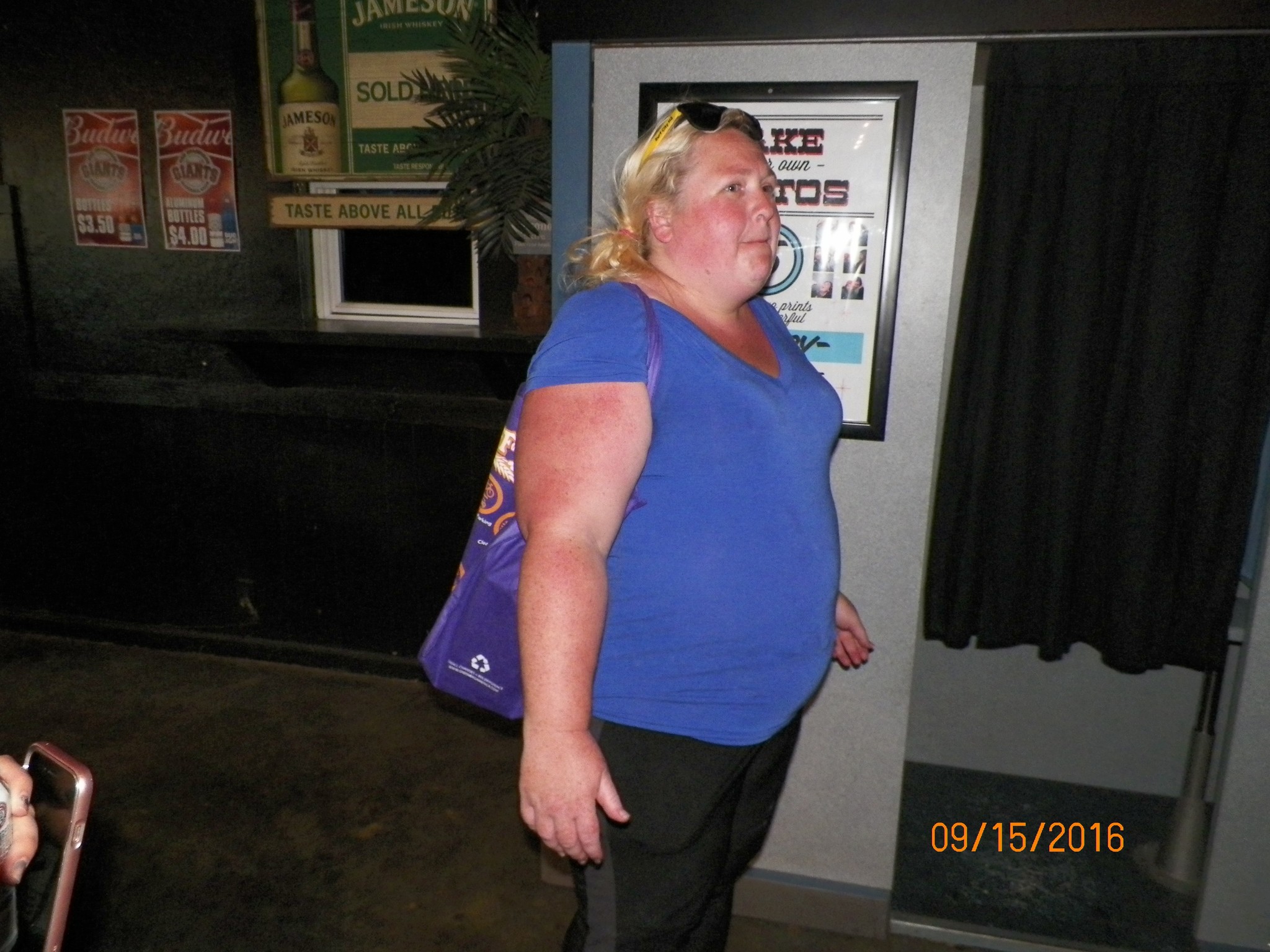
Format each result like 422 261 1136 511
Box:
636 101 763 171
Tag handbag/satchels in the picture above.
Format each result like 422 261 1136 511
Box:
420 274 664 719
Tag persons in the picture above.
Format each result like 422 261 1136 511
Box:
0 754 39 884
519 103 879 952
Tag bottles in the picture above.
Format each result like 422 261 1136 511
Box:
278 3 342 174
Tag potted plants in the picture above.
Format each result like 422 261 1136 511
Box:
401 1 550 333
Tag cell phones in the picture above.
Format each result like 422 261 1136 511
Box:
0 744 94 952
